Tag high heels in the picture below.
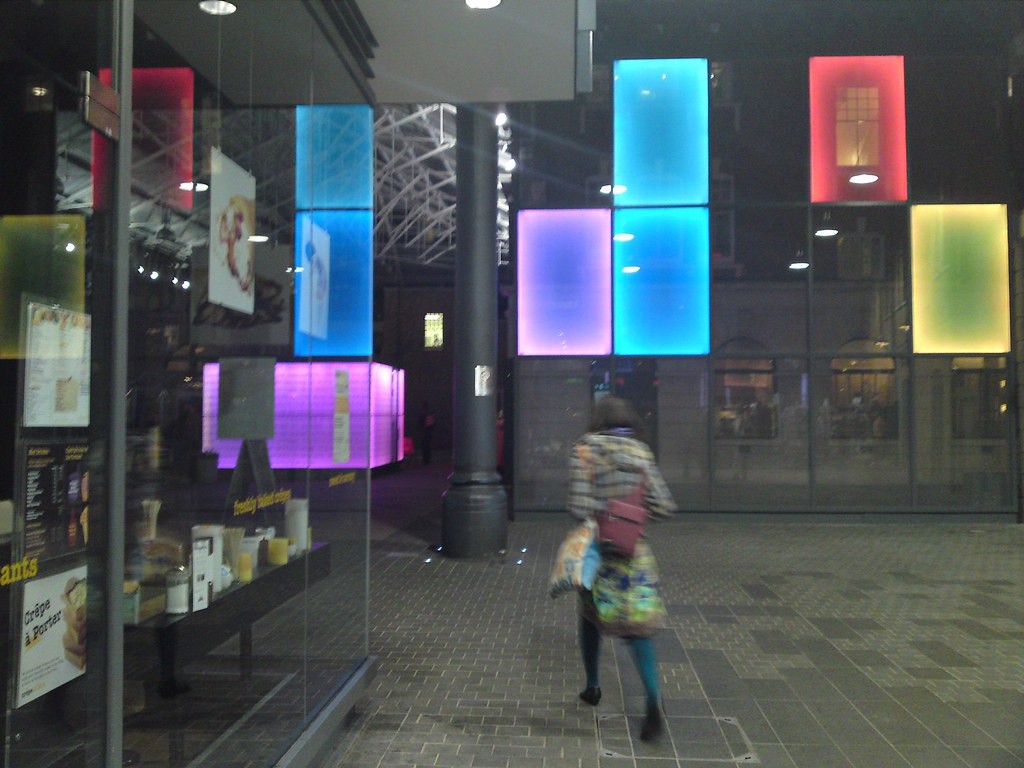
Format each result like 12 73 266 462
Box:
641 711 663 742
580 686 601 705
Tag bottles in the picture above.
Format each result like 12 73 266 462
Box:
258 537 268 568
165 563 189 613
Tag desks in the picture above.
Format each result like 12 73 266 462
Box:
77 537 330 768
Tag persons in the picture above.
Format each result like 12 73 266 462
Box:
822 393 897 439
714 393 778 440
119 383 196 728
422 414 435 465
565 398 675 743
613 373 658 425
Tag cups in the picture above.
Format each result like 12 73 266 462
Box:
241 538 257 567
307 527 312 550
222 573 231 589
285 499 309 551
268 539 288 565
239 553 252 582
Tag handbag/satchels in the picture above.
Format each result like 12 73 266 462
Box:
548 519 600 600
600 497 646 558
